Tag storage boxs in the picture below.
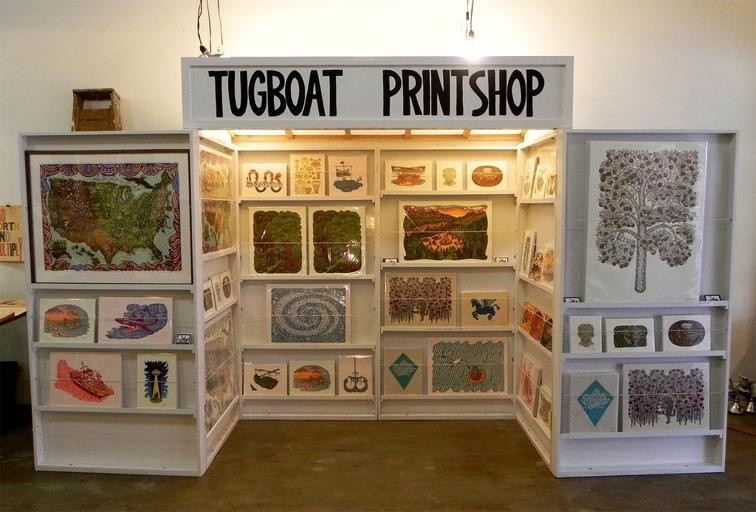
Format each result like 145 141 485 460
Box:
71 87 123 131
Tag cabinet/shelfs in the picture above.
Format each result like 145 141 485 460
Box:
234 146 377 421
377 145 516 420
513 131 560 478
193 130 240 478
17 131 202 478
559 130 737 477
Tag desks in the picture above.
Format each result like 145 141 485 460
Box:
0 299 27 326
